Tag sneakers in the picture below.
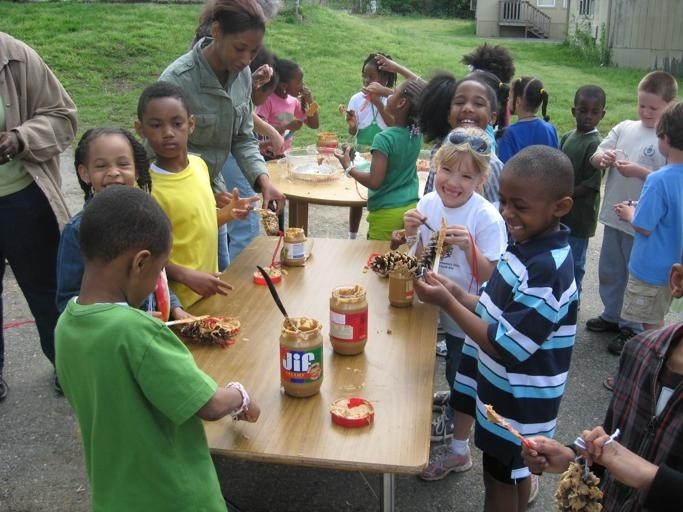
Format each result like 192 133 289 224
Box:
436 339 448 357
417 446 473 481
431 418 455 443
600 374 622 393
605 328 637 356
584 316 619 333
528 473 540 504
432 389 451 412
50 369 65 396
0 378 8 401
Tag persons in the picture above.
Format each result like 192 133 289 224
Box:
248 47 278 107
258 59 318 160
247 64 285 154
139 0 286 272
0 30 76 402
56 126 198 324
132 84 260 306
53 186 261 511
332 42 683 512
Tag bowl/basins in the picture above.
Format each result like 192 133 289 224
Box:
284 147 346 182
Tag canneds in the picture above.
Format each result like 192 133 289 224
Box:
388 272 415 308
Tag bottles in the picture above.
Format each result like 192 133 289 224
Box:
387 268 416 308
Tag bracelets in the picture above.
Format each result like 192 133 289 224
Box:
225 382 249 419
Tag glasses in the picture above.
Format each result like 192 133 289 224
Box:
447 132 489 153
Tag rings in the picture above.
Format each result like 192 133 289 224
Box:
9 158 13 161
7 154 10 158
